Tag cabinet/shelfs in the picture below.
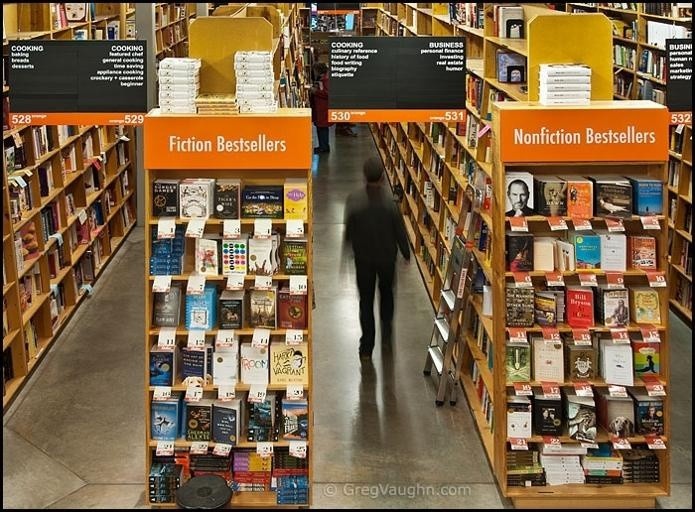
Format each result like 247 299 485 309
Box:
3 3 692 509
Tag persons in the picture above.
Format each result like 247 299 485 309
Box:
311 62 330 155
335 123 358 137
344 157 411 361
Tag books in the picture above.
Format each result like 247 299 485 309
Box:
365 4 693 488
2 3 226 398
146 3 314 506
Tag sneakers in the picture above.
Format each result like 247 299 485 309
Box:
314 147 330 154
359 344 372 360
348 123 356 127
335 129 357 137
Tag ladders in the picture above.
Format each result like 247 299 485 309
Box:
423 183 482 406
